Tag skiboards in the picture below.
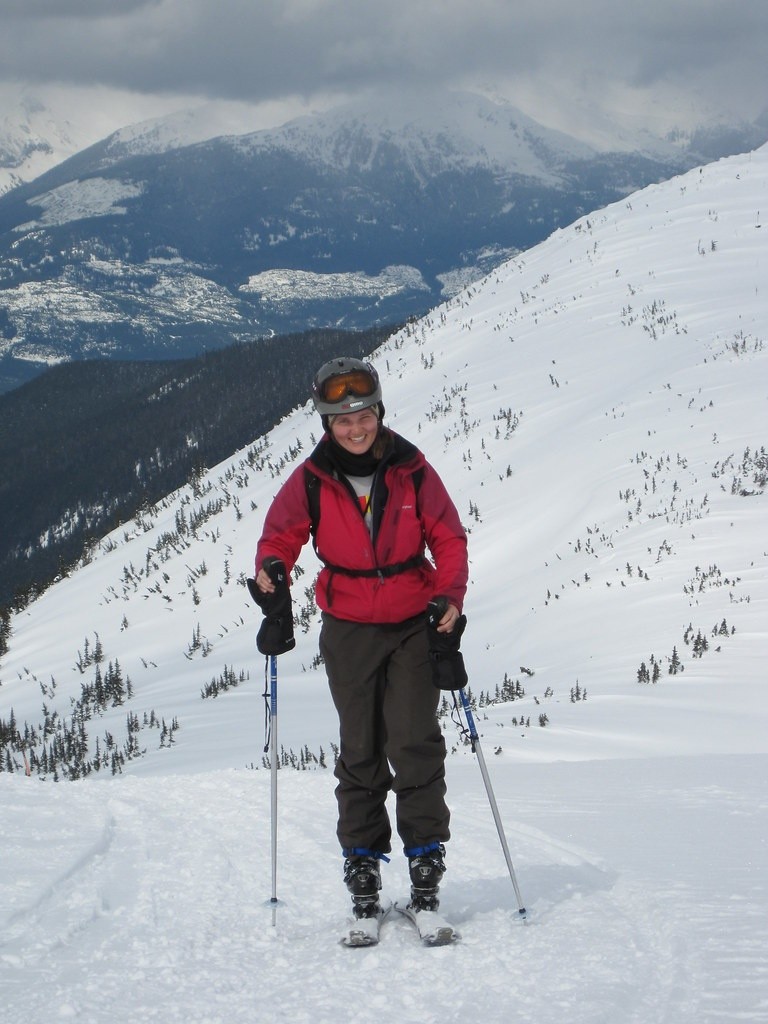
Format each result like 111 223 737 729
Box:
338 893 464 948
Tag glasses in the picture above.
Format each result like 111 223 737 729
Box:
319 369 378 404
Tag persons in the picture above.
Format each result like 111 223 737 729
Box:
254 356 467 918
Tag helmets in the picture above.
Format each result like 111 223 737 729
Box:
310 356 383 415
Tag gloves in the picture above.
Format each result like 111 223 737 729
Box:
423 595 468 691
247 556 296 656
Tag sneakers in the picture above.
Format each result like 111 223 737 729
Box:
408 843 447 888
341 855 383 895
